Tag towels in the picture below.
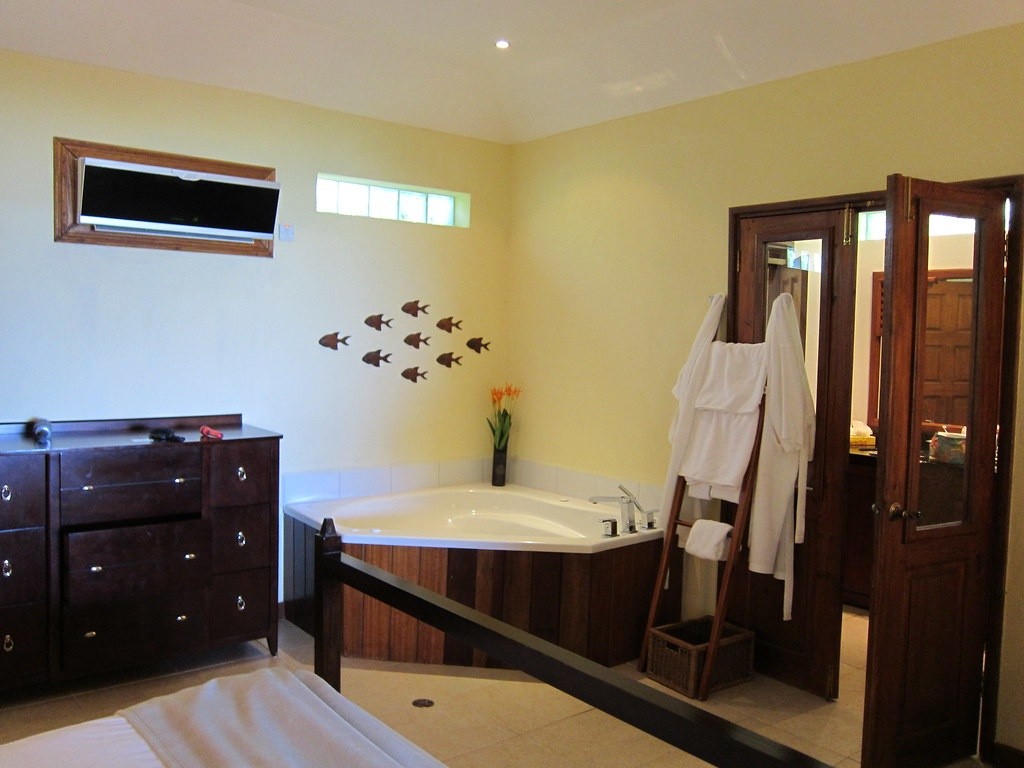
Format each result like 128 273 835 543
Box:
684 518 735 564
657 289 772 507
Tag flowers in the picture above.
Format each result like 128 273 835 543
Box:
485 380 523 450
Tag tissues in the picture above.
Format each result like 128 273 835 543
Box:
850 419 876 447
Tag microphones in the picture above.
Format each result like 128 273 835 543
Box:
33 418 52 446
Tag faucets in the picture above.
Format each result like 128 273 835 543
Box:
587 494 640 534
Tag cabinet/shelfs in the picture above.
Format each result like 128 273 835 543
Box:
1 436 279 713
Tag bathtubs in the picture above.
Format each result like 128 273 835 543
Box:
280 477 685 679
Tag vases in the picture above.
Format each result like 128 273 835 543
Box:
491 442 508 487
